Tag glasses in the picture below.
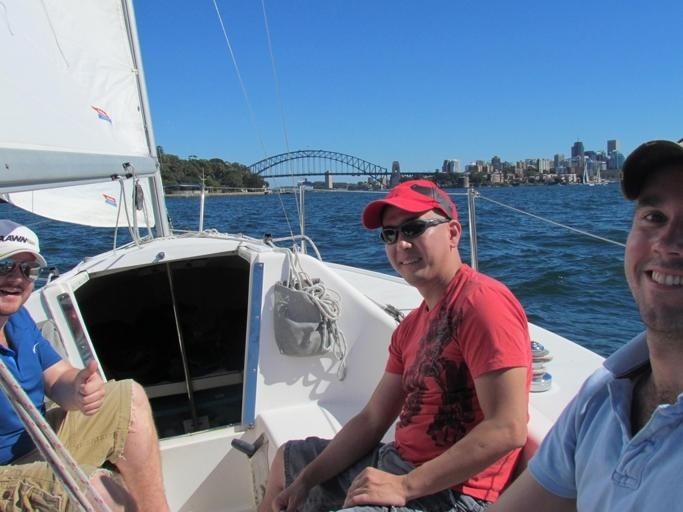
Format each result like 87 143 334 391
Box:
377 218 451 245
0 258 42 282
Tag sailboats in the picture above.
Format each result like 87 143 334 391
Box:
1 1 608 511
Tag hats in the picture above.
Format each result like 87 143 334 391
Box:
362 179 459 229
621 138 683 200
0 219 47 269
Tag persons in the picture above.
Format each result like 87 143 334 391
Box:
254 180 532 512
0 216 173 512
484 141 682 512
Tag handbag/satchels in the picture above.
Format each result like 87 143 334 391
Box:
274 279 335 356
